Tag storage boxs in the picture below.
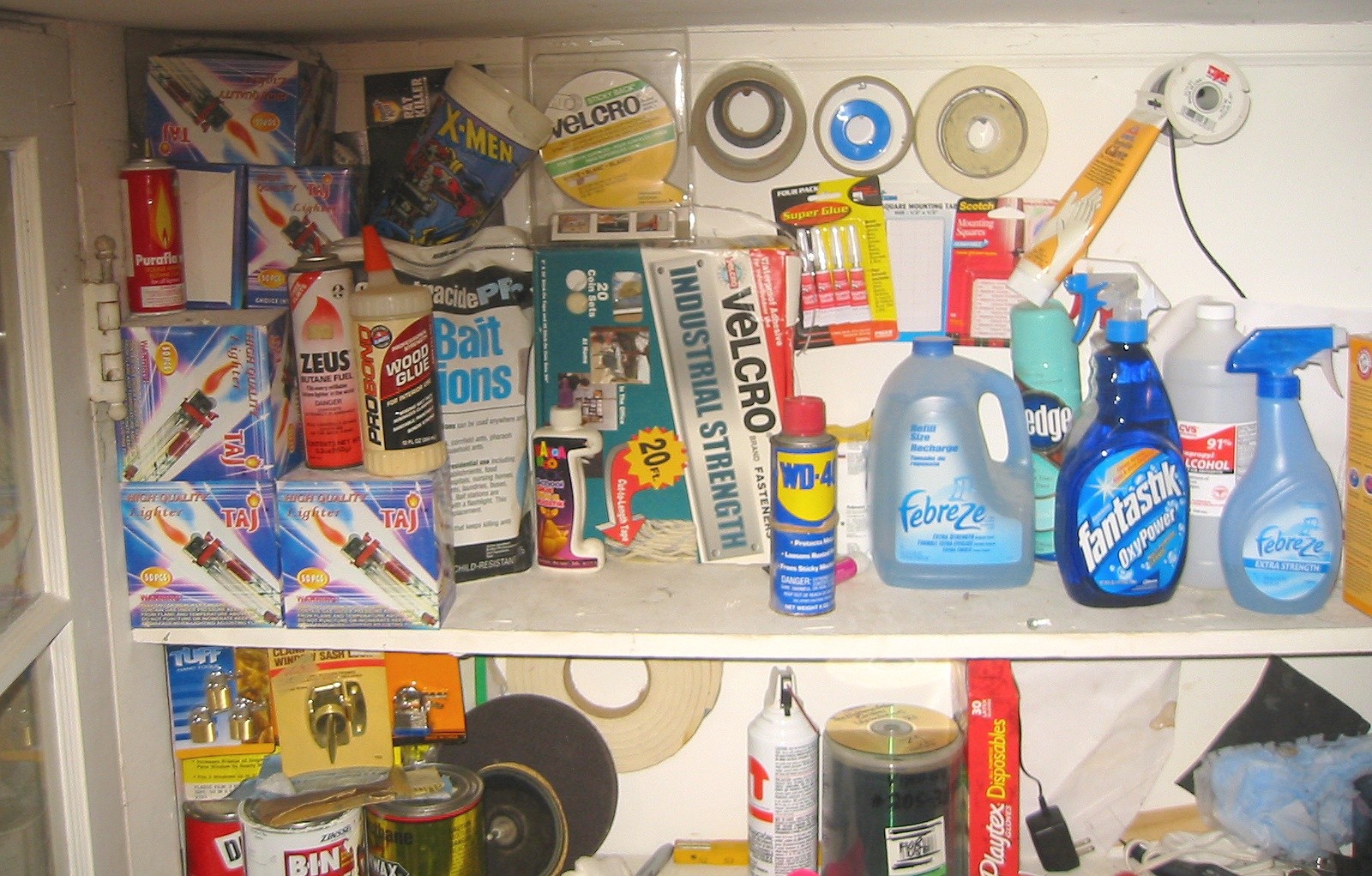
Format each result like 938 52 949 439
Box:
948 659 1021 876
1342 332 1372 619
121 42 802 629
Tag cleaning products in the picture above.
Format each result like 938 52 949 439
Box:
1219 323 1350 617
1053 271 1192 608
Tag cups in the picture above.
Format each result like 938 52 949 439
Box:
375 60 553 268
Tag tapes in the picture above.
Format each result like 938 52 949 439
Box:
942 92 1023 176
820 83 908 170
692 57 806 183
813 75 915 176
830 99 891 160
713 80 784 148
913 65 1049 201
504 657 721 777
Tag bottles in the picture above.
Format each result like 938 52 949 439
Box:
352 224 449 474
1012 298 1083 561
867 335 1035 589
768 395 836 616
286 236 365 472
120 138 190 314
746 666 820 875
1163 302 1260 591
532 376 605 572
